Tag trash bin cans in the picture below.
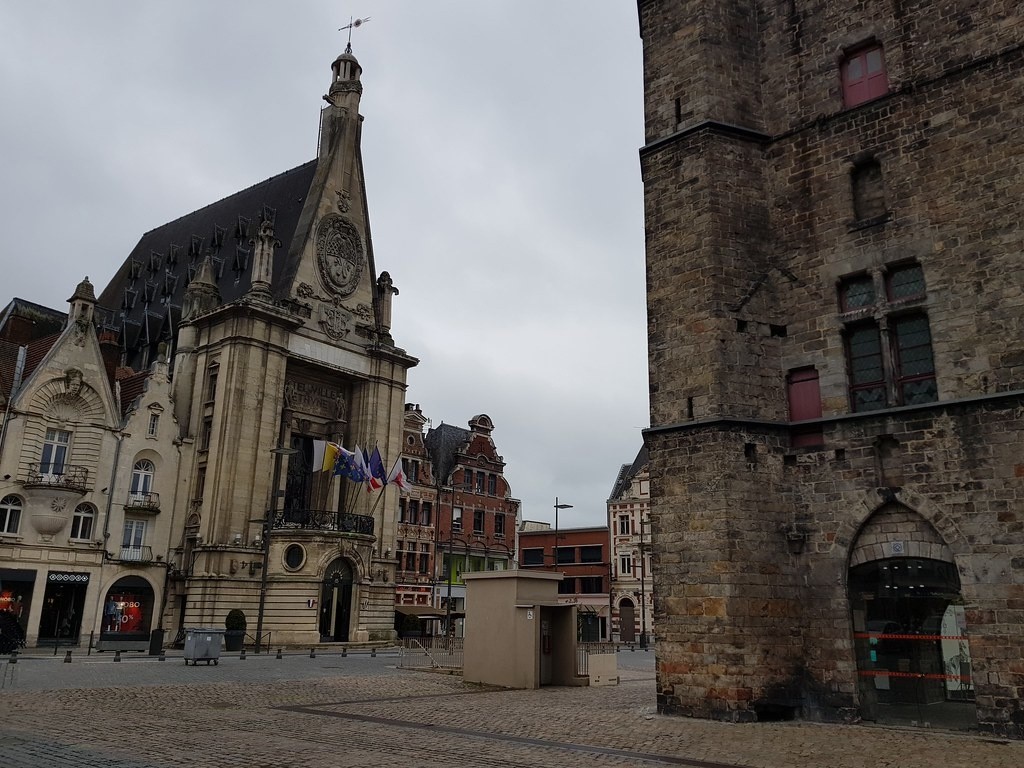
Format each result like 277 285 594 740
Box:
183 627 227 665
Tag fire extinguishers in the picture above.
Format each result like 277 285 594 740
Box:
544 635 552 655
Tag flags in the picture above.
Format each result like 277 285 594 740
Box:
366 446 387 492
314 439 381 493
390 455 414 494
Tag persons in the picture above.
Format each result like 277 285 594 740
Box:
114 596 125 632
8 596 24 619
105 596 118 631
